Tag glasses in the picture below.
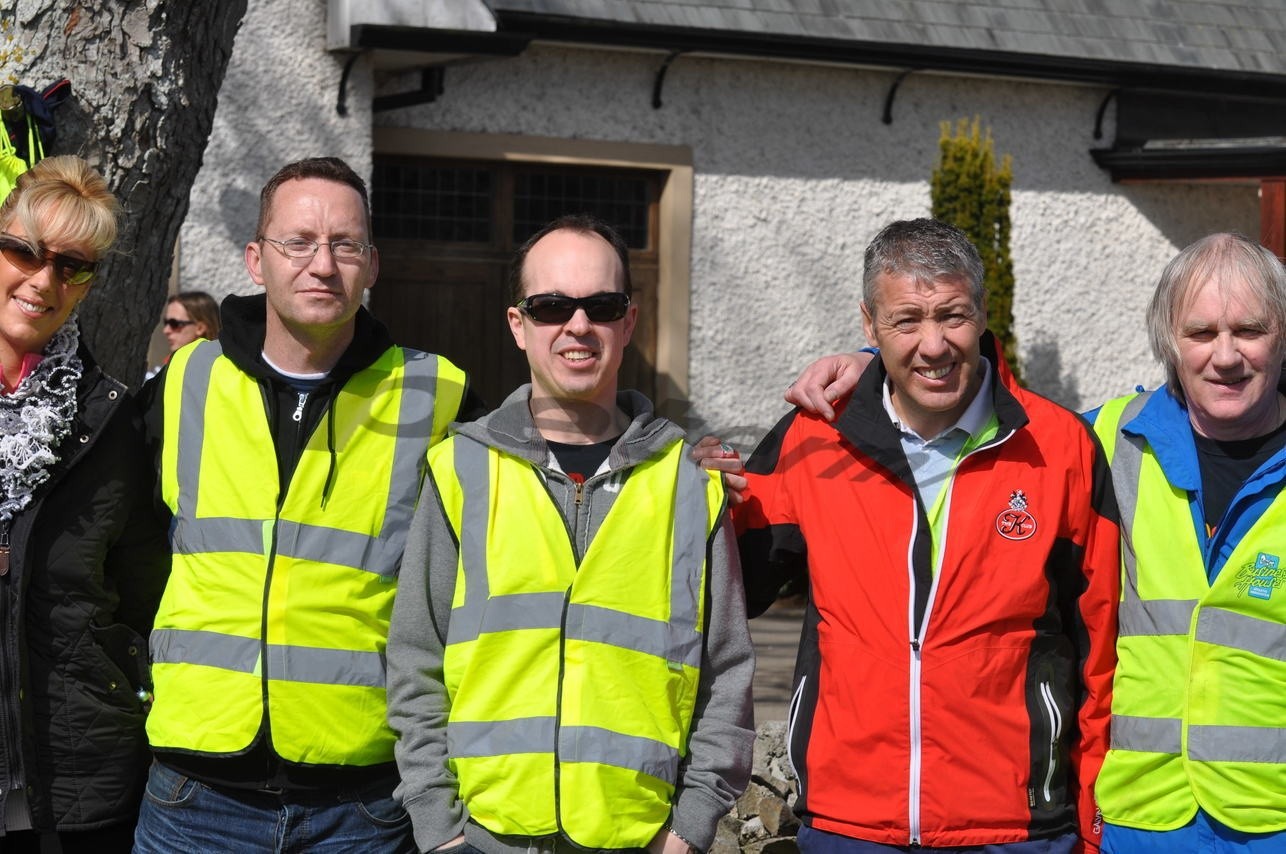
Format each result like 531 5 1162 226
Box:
164 318 193 330
254 236 373 259
516 292 631 324
0 232 101 286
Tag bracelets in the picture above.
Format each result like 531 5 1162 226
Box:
662 822 698 850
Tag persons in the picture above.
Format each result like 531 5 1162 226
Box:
131 156 493 854
0 155 159 854
782 231 1286 854
387 215 757 854
729 218 1120 854
145 291 222 383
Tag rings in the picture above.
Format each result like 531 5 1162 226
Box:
718 442 735 458
